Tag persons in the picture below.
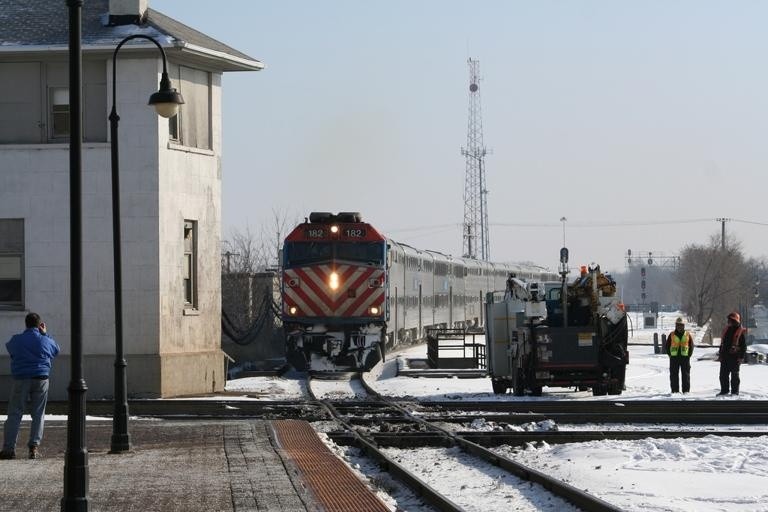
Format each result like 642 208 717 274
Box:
0 312 60 459
717 312 746 395
665 317 694 395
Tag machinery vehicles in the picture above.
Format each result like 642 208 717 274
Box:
476 266 630 397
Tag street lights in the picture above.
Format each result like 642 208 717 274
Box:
112 28 192 457
559 214 567 248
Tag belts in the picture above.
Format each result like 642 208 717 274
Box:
12 374 49 381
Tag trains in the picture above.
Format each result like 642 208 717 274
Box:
278 210 570 379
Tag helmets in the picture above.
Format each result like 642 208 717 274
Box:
727 312 740 325
676 317 684 325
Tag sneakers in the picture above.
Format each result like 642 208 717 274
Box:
0 448 15 460
27 448 43 459
